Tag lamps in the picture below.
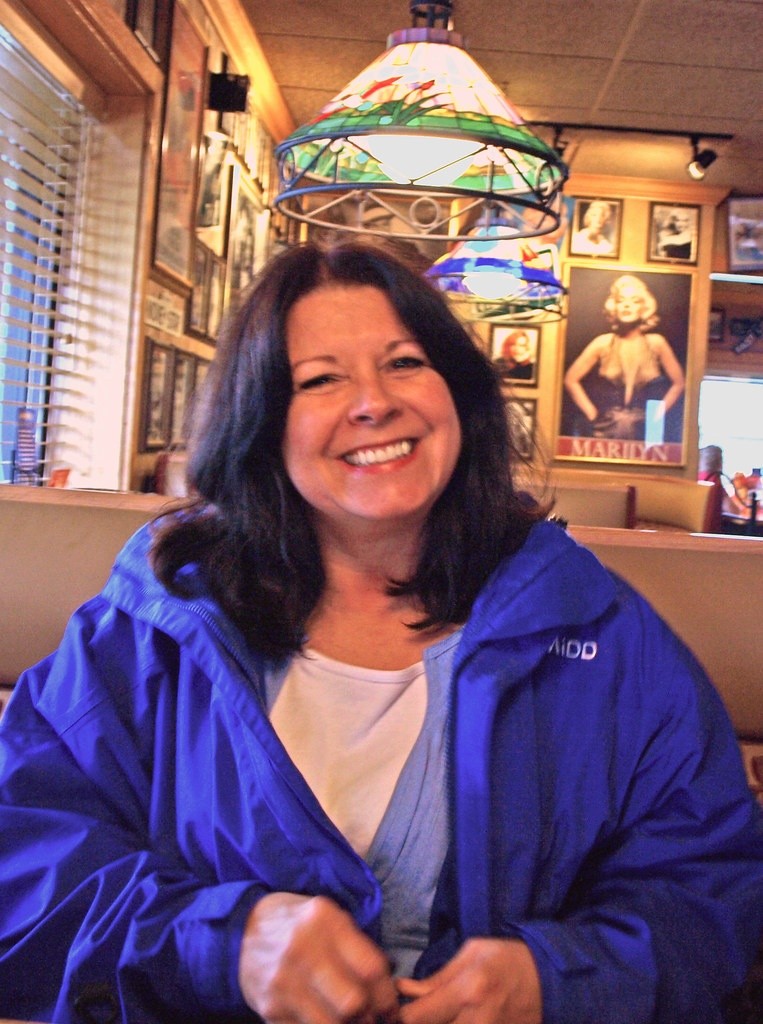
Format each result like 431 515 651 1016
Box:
688 148 717 179
272 0 570 242
424 208 569 306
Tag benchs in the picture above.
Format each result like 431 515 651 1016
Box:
508 464 720 534
0 485 763 802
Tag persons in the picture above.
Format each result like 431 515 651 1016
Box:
695 443 763 522
0 236 763 1024
467 199 702 448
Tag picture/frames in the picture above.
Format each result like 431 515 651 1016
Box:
221 55 304 248
150 0 212 300
551 261 699 467
646 201 702 266
503 396 537 461
488 322 542 388
132 0 170 62
709 307 726 342
726 192 763 273
301 190 460 263
185 238 224 346
568 194 624 259
224 165 271 339
138 336 212 451
